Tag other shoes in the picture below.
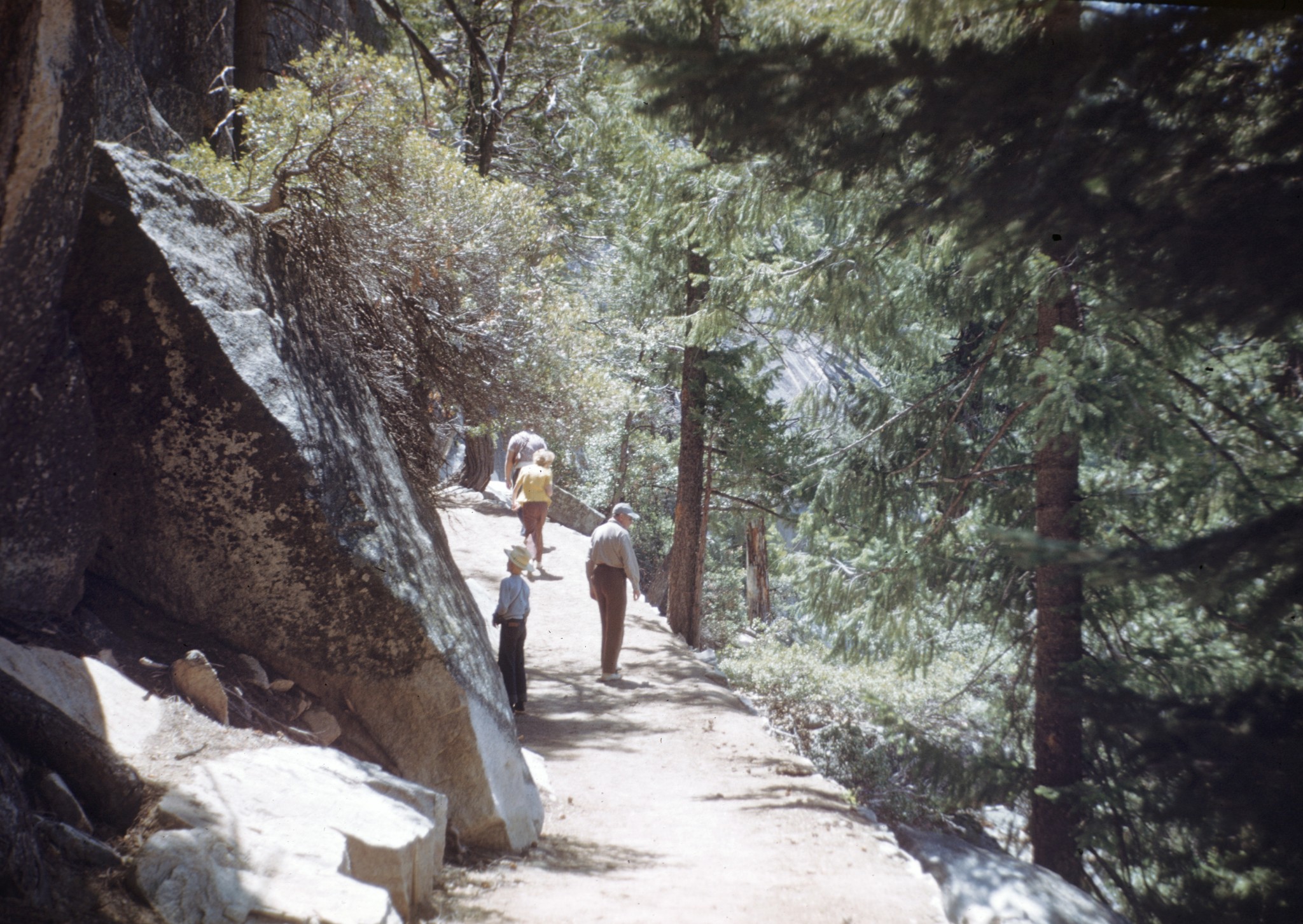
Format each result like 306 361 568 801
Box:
601 671 622 681
537 561 542 570
512 703 525 714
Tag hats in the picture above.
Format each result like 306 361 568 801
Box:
613 503 640 519
504 547 535 573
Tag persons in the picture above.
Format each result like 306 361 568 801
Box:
505 419 547 544
512 447 555 574
585 502 640 682
493 545 535 714
